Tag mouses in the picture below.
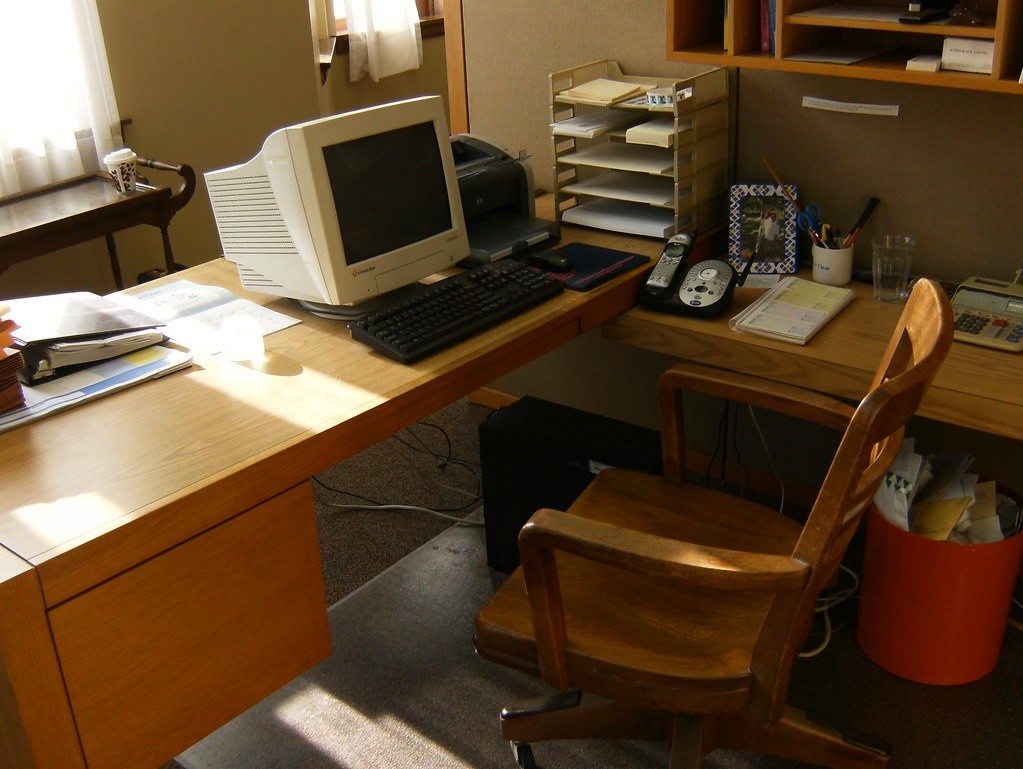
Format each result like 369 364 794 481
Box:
530 250 574 273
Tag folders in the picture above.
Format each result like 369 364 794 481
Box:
0 291 170 387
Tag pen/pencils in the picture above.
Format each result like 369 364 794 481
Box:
809 228 861 249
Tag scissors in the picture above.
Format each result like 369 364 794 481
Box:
798 205 821 235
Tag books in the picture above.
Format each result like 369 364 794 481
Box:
760 0 776 55
729 277 857 346
724 0 729 50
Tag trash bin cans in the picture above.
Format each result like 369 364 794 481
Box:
856 474 1023 687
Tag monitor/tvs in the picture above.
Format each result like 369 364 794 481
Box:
203 95 470 313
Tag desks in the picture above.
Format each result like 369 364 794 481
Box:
0 196 1023 769
0 156 198 293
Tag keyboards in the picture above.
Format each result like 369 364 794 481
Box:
349 259 565 364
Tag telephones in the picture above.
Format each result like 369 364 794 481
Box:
638 231 757 319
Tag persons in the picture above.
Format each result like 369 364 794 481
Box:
751 208 780 258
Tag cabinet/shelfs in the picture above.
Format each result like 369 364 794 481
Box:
547 58 740 250
665 0 1023 96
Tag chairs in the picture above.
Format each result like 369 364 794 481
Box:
472 276 956 769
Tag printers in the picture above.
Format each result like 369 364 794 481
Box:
449 133 562 271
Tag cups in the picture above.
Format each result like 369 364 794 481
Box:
103 148 137 194
870 234 915 303
811 237 854 286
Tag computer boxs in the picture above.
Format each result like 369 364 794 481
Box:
479 395 665 574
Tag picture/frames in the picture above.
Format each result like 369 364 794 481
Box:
729 185 797 275
